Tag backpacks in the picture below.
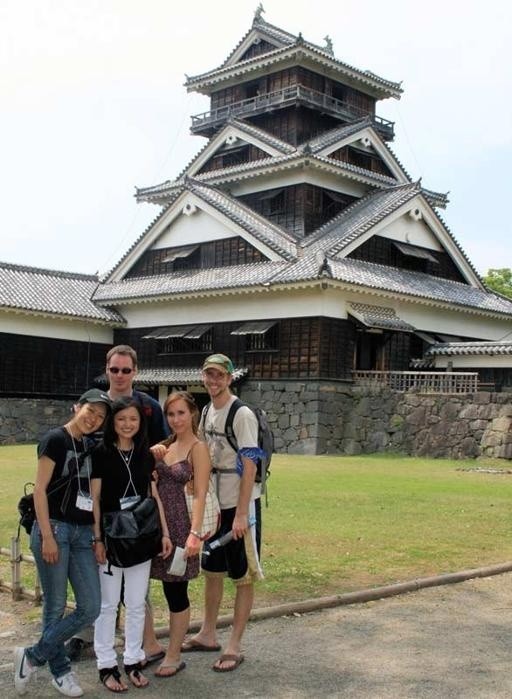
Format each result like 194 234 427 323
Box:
203 400 275 508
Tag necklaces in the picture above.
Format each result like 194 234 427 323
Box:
115 442 134 462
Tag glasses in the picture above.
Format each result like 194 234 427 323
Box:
108 366 135 374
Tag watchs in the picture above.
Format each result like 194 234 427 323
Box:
190 530 202 538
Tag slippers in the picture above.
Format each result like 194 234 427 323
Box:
213 654 243 672
141 650 165 666
154 660 186 678
180 638 221 652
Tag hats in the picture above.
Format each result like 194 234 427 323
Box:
202 353 234 375
71 389 113 415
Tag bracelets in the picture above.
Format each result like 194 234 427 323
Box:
163 535 171 538
95 539 102 542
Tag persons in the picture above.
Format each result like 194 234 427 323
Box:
89 397 171 693
181 354 275 673
150 390 222 677
75 345 167 669
13 388 113 698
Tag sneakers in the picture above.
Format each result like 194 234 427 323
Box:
65 638 93 661
51 670 84 697
14 647 37 696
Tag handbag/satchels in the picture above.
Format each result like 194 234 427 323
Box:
184 441 222 541
17 438 100 535
100 445 163 568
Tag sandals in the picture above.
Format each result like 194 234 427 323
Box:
124 662 150 689
99 666 127 692
